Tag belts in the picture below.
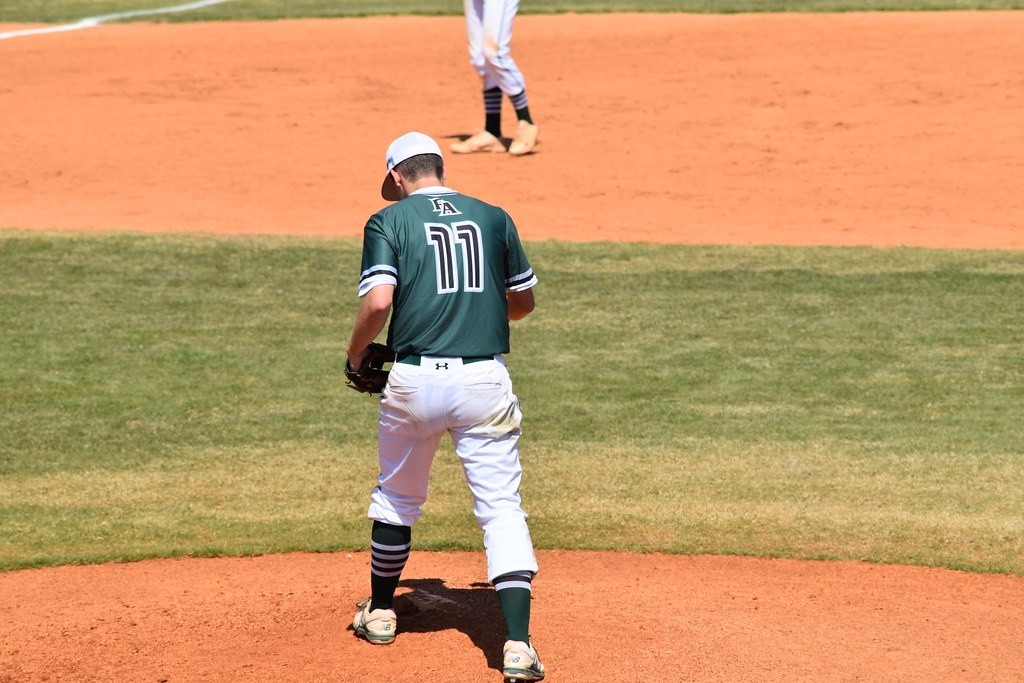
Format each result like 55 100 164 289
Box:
396 353 494 367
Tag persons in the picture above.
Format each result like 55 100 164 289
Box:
446 1 540 158
342 130 554 683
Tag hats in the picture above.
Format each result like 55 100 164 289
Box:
381 132 443 201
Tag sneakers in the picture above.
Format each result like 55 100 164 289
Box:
450 130 508 154
502 640 545 682
508 119 538 154
353 597 397 645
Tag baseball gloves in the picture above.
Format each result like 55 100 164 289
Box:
344 341 400 394
